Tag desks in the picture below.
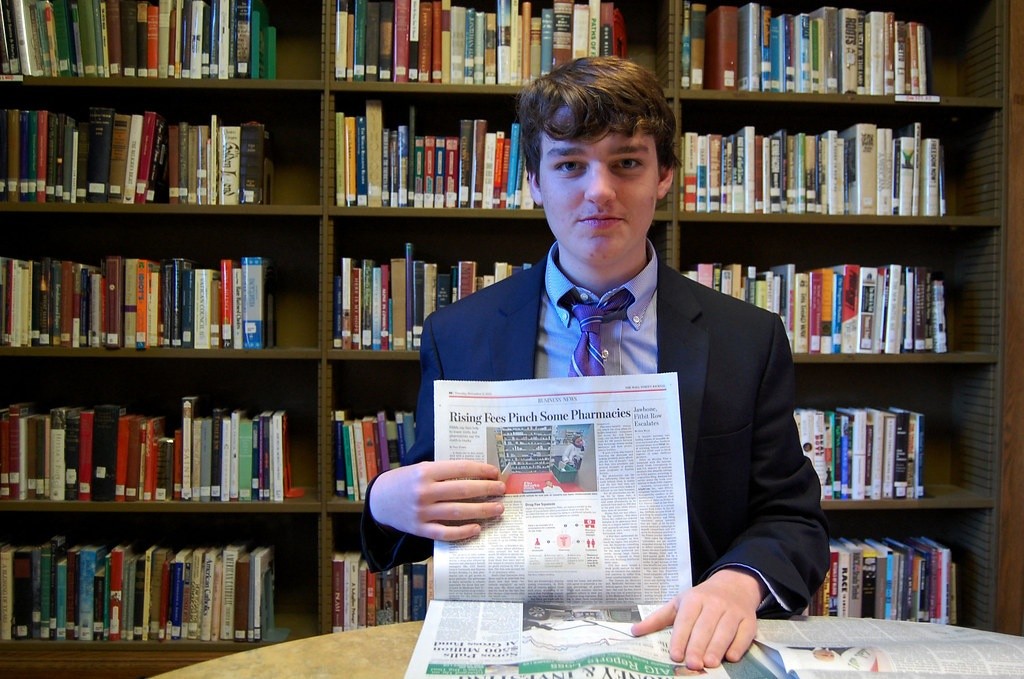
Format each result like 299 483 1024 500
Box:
150 614 1024 679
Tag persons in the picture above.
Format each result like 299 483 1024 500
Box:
788 647 853 662
358 56 833 671
561 436 585 471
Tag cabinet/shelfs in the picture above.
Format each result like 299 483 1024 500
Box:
0 0 1024 679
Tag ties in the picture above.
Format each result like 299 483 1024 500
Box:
561 289 634 378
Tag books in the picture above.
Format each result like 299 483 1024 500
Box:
790 405 927 500
678 118 949 218
0 246 277 351
0 397 285 503
334 405 422 503
335 97 547 210
332 0 617 86
681 260 955 355
0 0 280 82
0 533 271 642
331 549 436 634
681 0 930 95
0 107 274 205
332 240 534 352
800 536 959 627
